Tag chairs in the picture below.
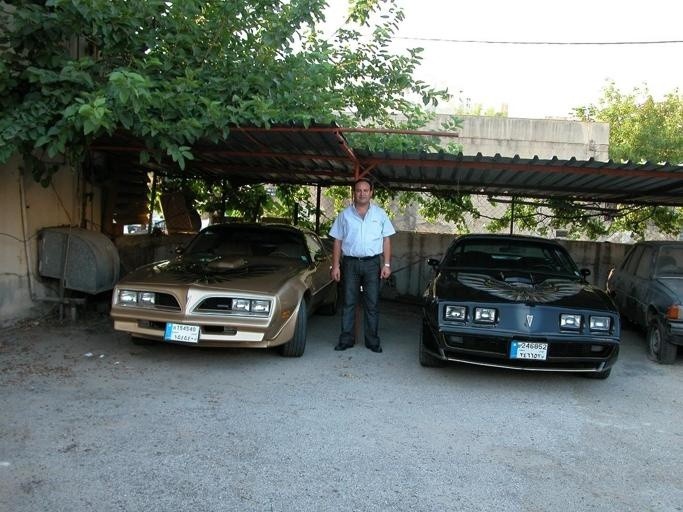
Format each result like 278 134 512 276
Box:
459 249 555 272
654 255 681 277
210 237 309 263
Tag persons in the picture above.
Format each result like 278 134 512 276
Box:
325 175 394 353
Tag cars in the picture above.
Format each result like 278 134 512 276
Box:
605 241 683 364
109 222 341 357
419 234 621 379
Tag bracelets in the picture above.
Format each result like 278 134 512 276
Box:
383 262 390 267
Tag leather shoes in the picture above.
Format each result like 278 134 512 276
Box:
334 341 382 353
